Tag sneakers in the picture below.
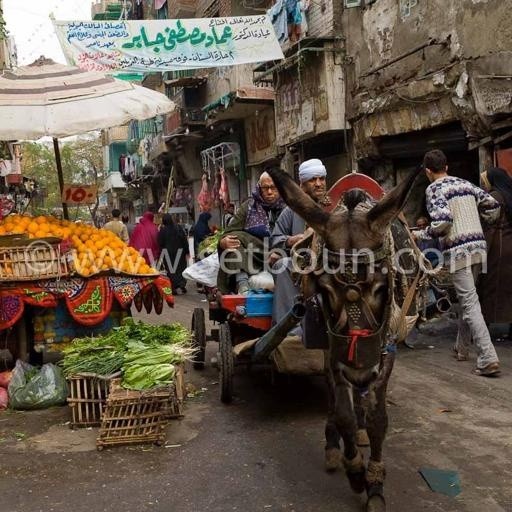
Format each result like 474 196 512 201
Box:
448 342 470 361
472 361 501 376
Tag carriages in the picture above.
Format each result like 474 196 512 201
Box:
192 166 432 512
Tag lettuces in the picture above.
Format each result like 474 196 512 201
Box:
121 340 185 391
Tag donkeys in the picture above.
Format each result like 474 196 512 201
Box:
265 162 422 512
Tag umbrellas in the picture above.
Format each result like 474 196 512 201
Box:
0 54 175 221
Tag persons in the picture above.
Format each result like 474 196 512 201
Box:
408 149 502 376
157 213 189 295
130 211 158 265
469 169 512 342
217 170 284 293
268 156 326 339
193 212 212 294
120 214 136 244
412 216 442 269
101 209 129 246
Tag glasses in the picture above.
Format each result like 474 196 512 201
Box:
258 184 277 192
